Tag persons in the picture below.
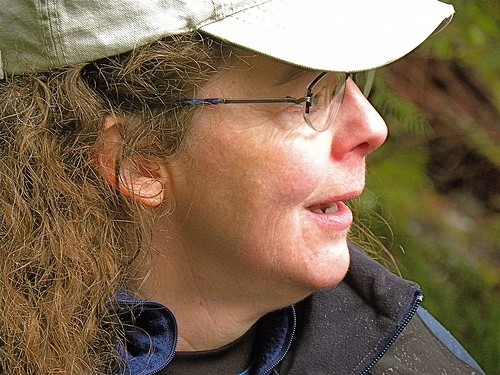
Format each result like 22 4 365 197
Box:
0 0 487 375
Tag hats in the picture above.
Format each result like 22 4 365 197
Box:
0 1 456 77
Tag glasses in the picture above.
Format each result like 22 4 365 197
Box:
124 67 376 132
